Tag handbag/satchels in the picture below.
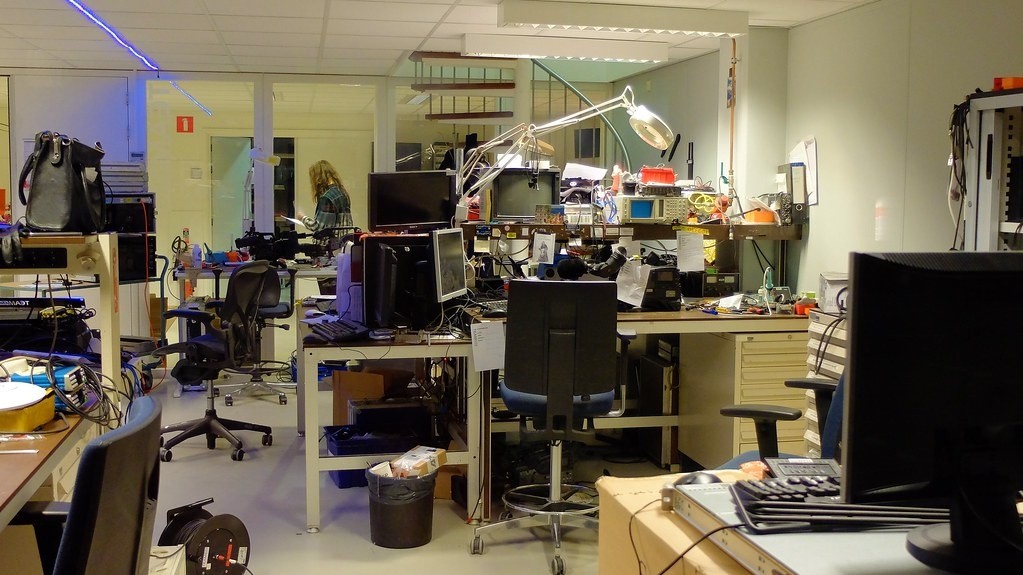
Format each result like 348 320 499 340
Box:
18 129 107 232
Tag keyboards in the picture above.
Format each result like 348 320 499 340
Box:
483 301 507 311
311 318 369 342
729 475 840 535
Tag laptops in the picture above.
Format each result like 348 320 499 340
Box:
482 309 507 318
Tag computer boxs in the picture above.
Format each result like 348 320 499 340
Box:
361 234 444 328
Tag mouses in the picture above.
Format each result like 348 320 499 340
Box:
302 333 328 344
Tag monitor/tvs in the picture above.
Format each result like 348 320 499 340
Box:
839 251 1023 575
479 167 560 222
429 228 467 302
367 170 457 231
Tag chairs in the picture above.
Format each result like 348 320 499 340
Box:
151 261 298 460
717 368 839 470
469 279 619 575
7 396 162 575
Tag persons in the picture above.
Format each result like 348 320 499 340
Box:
297 160 354 295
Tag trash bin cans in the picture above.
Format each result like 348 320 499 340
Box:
365 460 438 549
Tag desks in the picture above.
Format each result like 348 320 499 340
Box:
297 298 483 533
0 393 104 575
468 296 823 524
596 470 1023 575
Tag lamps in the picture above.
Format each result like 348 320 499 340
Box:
248 147 281 169
459 82 673 229
461 33 670 63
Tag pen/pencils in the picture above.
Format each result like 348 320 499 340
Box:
702 310 718 315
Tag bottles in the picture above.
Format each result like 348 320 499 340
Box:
191 244 202 270
